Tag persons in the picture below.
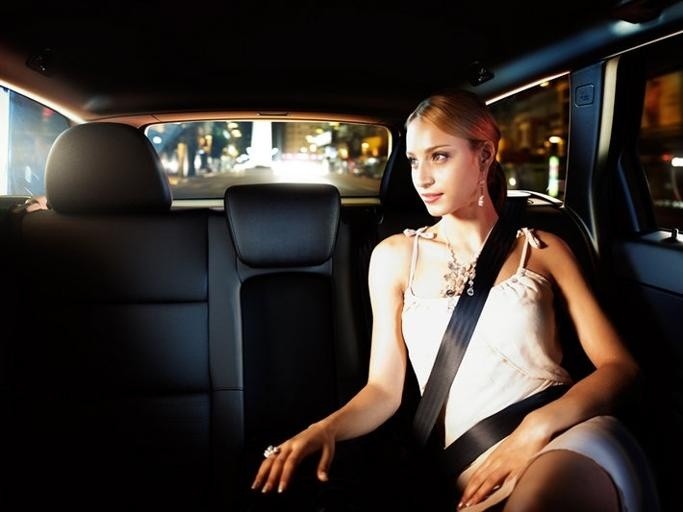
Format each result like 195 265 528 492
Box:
248 89 661 512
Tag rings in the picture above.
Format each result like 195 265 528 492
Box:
262 446 280 458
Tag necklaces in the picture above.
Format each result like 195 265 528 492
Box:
435 214 498 299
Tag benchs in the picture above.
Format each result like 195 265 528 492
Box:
1 123 647 496
